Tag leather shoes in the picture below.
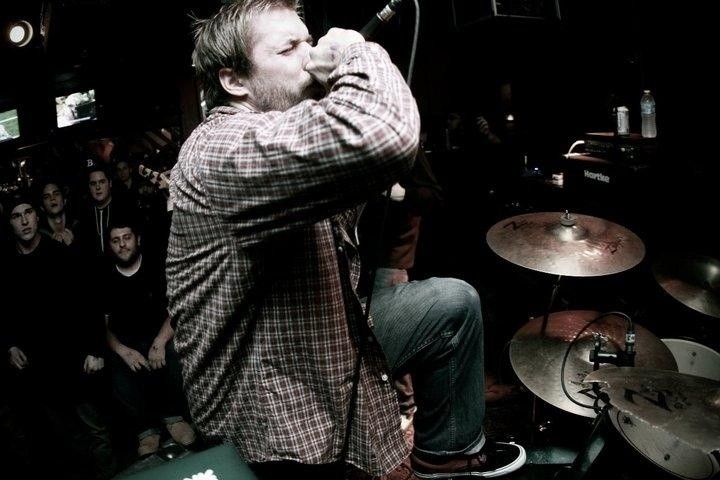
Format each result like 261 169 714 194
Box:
138 430 160 456
166 420 196 445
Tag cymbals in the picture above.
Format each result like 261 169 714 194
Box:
509 310 678 419
486 212 646 277
658 339 720 382
652 243 720 317
583 366 719 453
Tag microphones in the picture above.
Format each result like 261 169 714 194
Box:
359 0 402 41
625 324 635 352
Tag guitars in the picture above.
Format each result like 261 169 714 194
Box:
139 165 169 190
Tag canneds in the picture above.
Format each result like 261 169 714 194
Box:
612 105 630 137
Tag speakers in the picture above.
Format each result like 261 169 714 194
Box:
563 152 679 338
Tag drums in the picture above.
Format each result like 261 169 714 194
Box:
565 403 720 480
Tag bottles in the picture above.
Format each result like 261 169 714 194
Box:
640 90 657 138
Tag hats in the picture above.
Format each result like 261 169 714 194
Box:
3 193 30 220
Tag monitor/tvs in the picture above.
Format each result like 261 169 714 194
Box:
53 87 100 131
0 106 23 146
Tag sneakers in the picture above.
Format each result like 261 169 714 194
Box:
410 442 527 479
